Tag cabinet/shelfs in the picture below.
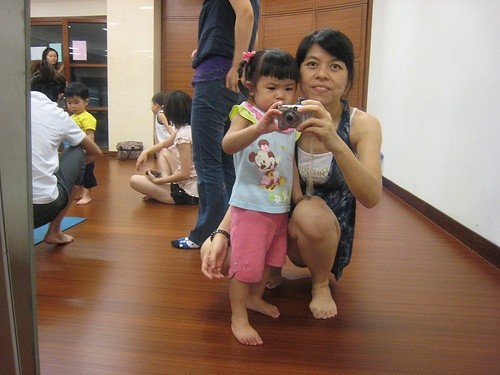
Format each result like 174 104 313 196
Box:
160 0 373 118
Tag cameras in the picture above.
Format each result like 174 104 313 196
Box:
278 105 315 129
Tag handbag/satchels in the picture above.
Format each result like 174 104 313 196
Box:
116 140 144 160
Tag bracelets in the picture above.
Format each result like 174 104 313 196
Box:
210 229 231 247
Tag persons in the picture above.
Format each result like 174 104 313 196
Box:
199 28 383 320
151 93 178 147
222 48 302 345
42 47 65 75
129 92 202 205
30 59 103 244
171 0 260 249
63 81 97 204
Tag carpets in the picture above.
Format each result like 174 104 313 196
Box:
33 216 87 246
